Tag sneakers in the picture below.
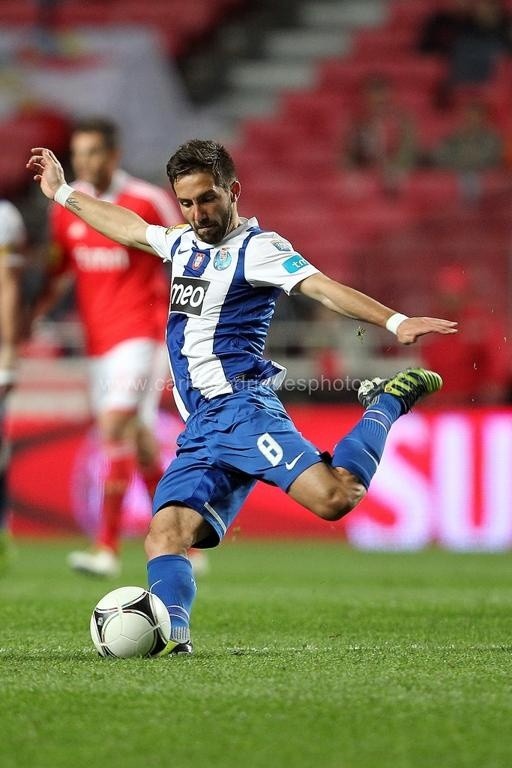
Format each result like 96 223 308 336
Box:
65 543 122 579
151 638 194 657
357 367 444 415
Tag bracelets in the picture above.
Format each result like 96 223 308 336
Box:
52 185 76 206
384 312 409 333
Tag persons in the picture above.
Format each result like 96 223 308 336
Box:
0 197 27 563
18 115 207 581
27 139 458 658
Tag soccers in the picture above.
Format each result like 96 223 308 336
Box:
90 587 171 659
